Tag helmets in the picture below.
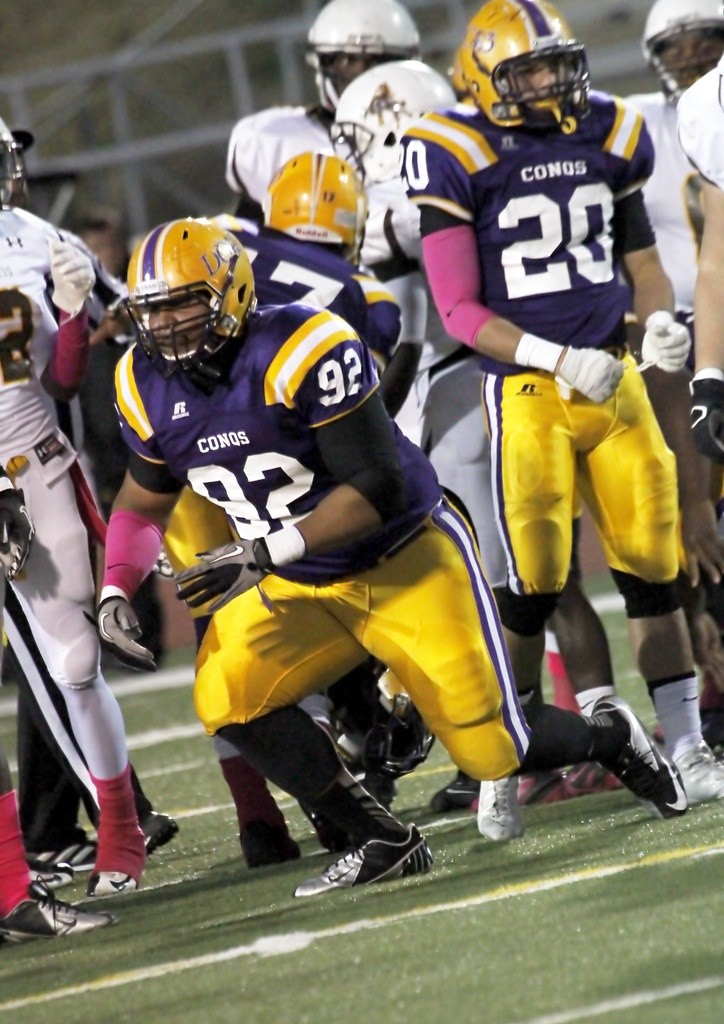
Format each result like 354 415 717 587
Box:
461 0 591 129
333 57 456 182
642 1 724 91
127 217 257 367
0 119 27 210
306 1 420 111
264 153 370 265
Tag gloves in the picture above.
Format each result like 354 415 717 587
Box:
689 378 724 464
97 596 159 672
1 488 37 579
639 310 690 371
46 240 95 316
554 346 623 403
174 536 276 613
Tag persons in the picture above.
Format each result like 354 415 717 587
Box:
0 112 180 944
89 218 689 897
148 0 724 868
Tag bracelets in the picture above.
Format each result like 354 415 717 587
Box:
515 331 564 374
264 524 306 568
690 367 724 383
644 309 672 328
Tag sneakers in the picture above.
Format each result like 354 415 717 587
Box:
476 777 525 844
291 822 433 896
563 763 623 798
0 812 179 944
677 744 724 804
241 821 299 867
470 759 565 810
593 694 688 819
431 772 478 810
